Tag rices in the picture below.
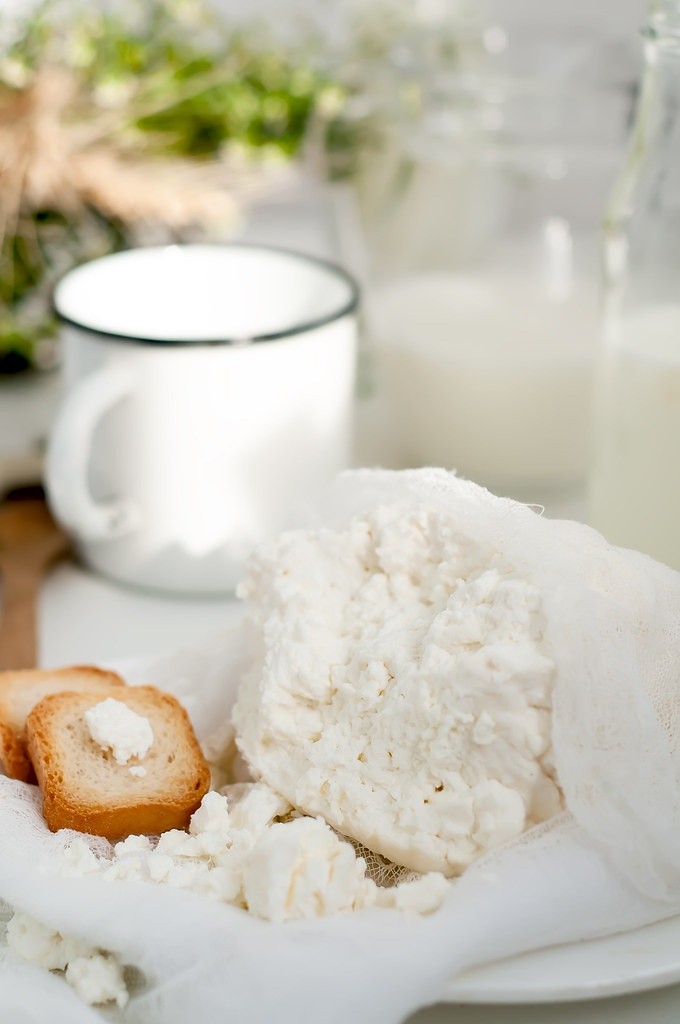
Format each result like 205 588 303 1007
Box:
0 466 585 1006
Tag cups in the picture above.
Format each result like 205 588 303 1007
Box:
48 240 358 601
